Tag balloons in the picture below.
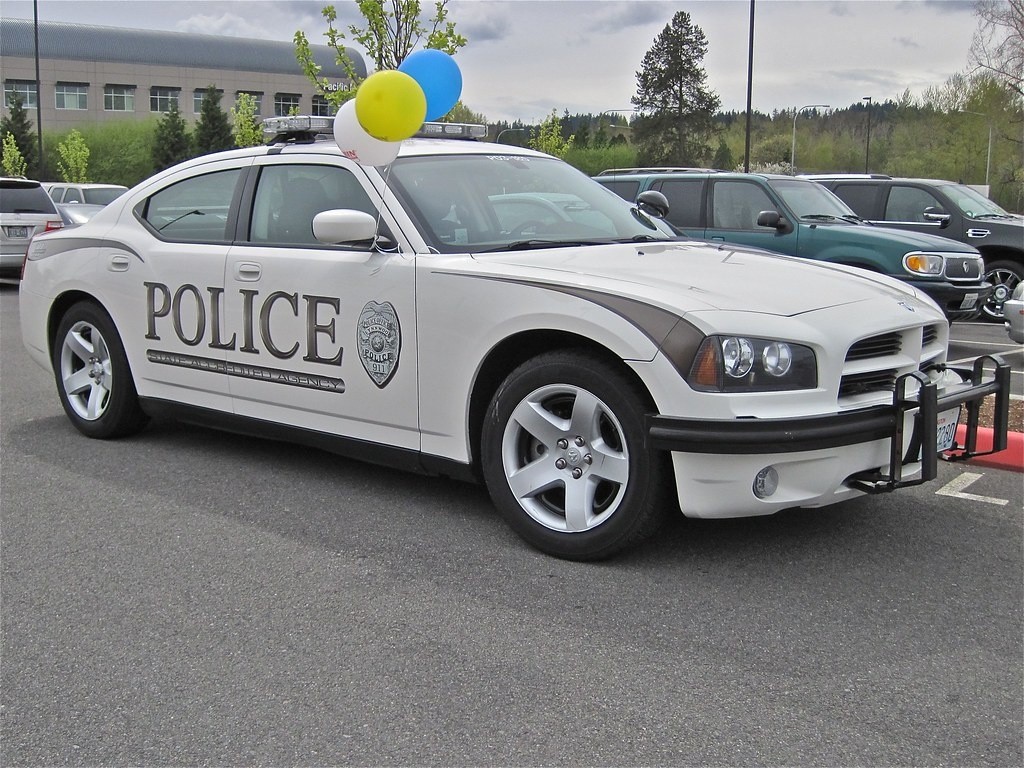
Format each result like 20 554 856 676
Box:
333 98 401 166
398 49 462 122
355 70 427 142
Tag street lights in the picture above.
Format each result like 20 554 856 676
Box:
791 104 831 174
496 128 524 143
959 109 992 185
600 109 636 135
863 97 871 172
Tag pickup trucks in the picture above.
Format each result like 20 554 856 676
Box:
40 181 129 224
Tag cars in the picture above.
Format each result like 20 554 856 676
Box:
1003 279 1024 344
488 193 693 240
18 115 965 562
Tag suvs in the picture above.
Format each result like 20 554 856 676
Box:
794 174 1024 323
591 167 993 327
0 175 62 270
